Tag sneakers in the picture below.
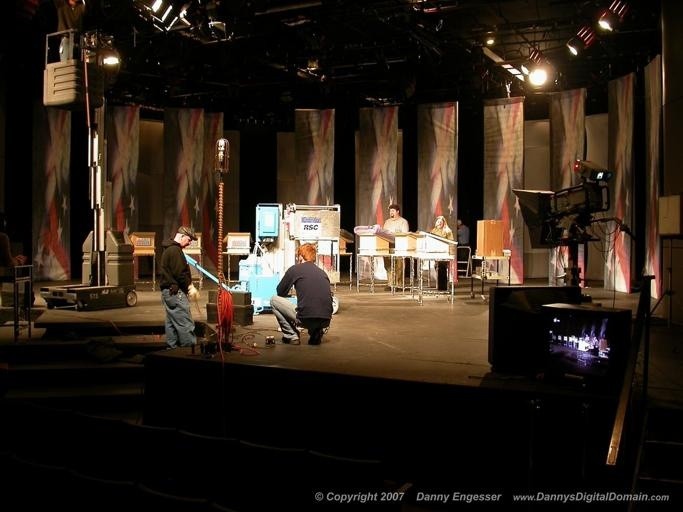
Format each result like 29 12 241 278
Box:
281 336 301 345
385 284 403 293
307 328 324 346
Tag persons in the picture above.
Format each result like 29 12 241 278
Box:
381 204 409 292
456 219 470 276
157 225 201 351
429 215 453 295
268 243 333 343
0 213 18 268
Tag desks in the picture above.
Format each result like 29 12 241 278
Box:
469 252 512 304
334 252 454 307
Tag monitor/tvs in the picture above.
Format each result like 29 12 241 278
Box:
538 302 632 394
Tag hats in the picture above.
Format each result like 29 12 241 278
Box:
177 224 199 241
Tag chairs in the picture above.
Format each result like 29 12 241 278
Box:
0 265 34 340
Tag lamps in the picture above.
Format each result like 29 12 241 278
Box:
565 0 627 57
520 48 544 75
